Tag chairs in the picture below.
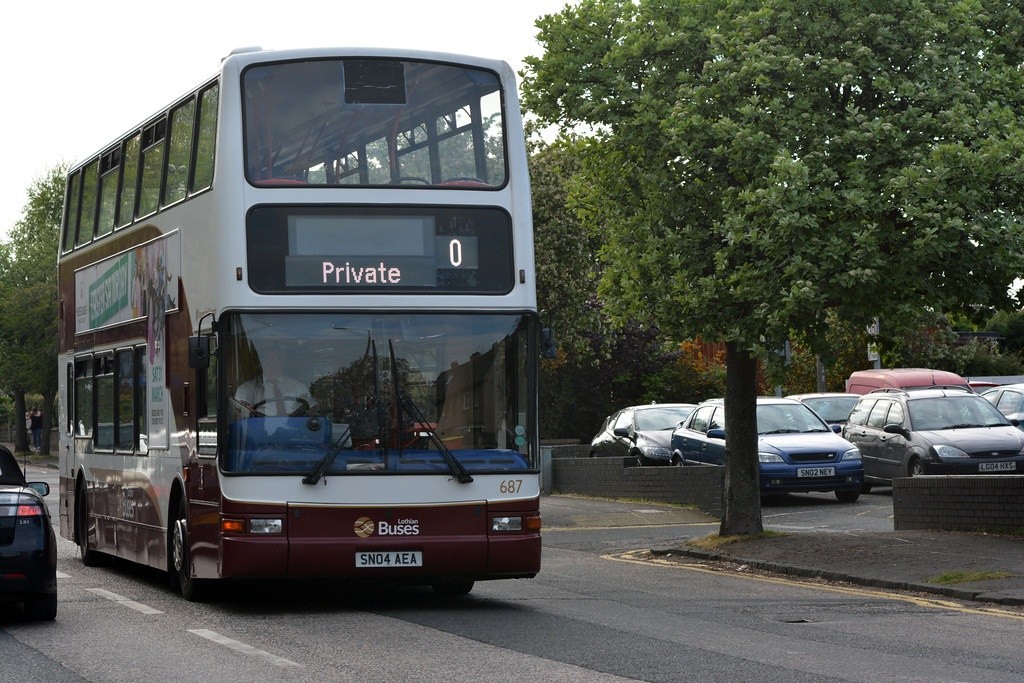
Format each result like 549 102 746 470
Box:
253 174 483 187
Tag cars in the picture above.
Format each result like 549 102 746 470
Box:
784 393 862 437
961 384 1024 434
587 403 699 467
0 444 58 623
670 396 866 504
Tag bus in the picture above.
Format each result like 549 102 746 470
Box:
59 45 541 614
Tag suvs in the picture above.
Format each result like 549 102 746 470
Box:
961 376 1024 395
840 388 1024 495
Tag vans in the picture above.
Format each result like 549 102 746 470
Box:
845 369 984 425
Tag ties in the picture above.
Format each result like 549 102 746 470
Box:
269 379 287 417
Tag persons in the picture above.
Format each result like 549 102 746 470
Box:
234 344 320 418
25 407 44 450
